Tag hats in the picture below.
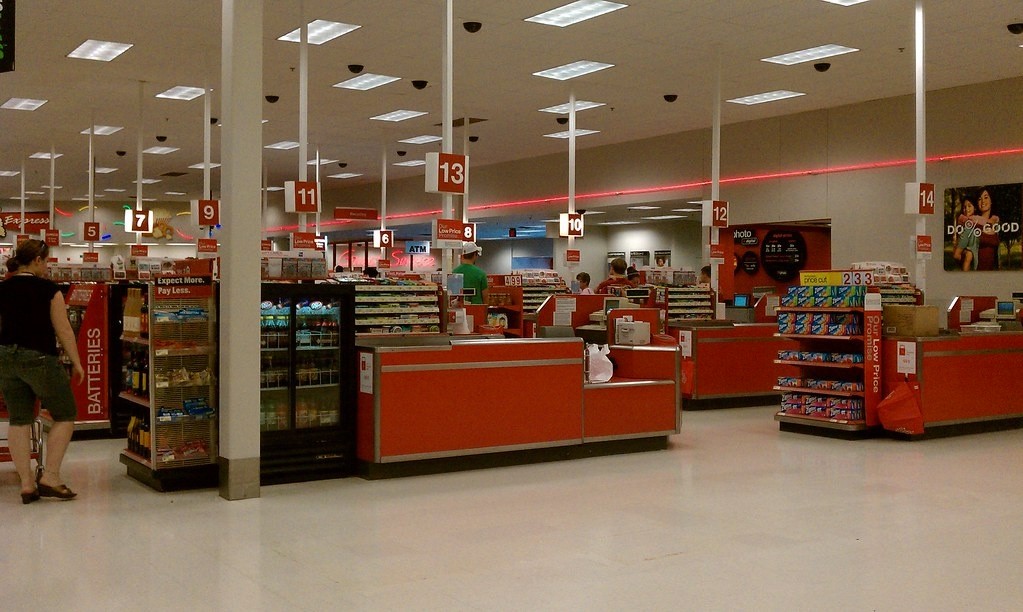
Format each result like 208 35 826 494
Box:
462 243 479 255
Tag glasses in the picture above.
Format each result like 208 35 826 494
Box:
40 240 47 251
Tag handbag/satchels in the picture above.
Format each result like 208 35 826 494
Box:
583 343 613 384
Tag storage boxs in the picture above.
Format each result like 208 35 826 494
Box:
777 285 880 421
883 305 940 336
618 322 651 345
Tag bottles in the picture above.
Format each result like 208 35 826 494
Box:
128 292 151 460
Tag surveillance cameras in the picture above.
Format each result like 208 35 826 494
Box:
556 118 569 124
397 151 406 156
463 22 482 34
339 163 347 168
357 244 362 247
468 136 479 142
116 151 126 156
814 63 831 72
348 65 363 74
156 136 167 142
210 118 217 124
265 96 279 103
410 80 428 90
576 210 587 214
663 95 678 102
1007 23 1023 34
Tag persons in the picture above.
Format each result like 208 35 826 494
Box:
451 243 489 324
576 272 595 295
700 266 711 285
0 239 85 504
597 259 640 295
953 187 1000 271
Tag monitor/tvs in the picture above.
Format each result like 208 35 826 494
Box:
733 294 749 306
603 297 640 320
995 300 1015 319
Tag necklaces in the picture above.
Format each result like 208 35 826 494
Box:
19 270 35 277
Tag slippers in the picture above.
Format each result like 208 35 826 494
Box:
37 483 77 498
20 491 39 504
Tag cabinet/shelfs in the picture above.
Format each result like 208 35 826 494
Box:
773 293 882 431
355 285 449 336
656 287 717 322
881 289 925 304
56 275 221 493
523 280 573 310
